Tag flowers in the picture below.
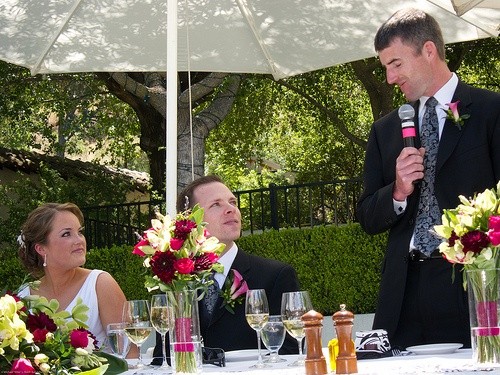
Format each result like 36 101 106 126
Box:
218 268 251 315
0 271 129 375
427 180 500 363
440 100 470 130
132 196 251 373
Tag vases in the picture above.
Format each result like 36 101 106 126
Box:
167 291 203 375
466 268 500 367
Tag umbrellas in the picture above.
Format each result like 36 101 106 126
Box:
1 0 500 214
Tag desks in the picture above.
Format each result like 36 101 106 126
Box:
120 350 500 375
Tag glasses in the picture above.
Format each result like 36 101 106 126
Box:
139 354 171 368
201 346 226 367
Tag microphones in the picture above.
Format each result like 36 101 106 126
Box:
397 104 422 185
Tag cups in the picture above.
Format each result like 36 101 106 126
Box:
107 322 132 358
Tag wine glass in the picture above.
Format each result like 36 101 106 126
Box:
150 295 171 371
281 291 313 366
261 315 287 363
121 299 154 371
245 290 272 369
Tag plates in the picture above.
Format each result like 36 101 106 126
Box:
225 349 270 359
405 343 463 354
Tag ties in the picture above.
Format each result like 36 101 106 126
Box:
203 269 220 313
412 96 443 259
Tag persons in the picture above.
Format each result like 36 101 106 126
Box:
160 175 301 355
356 7 500 350
15 202 140 359
